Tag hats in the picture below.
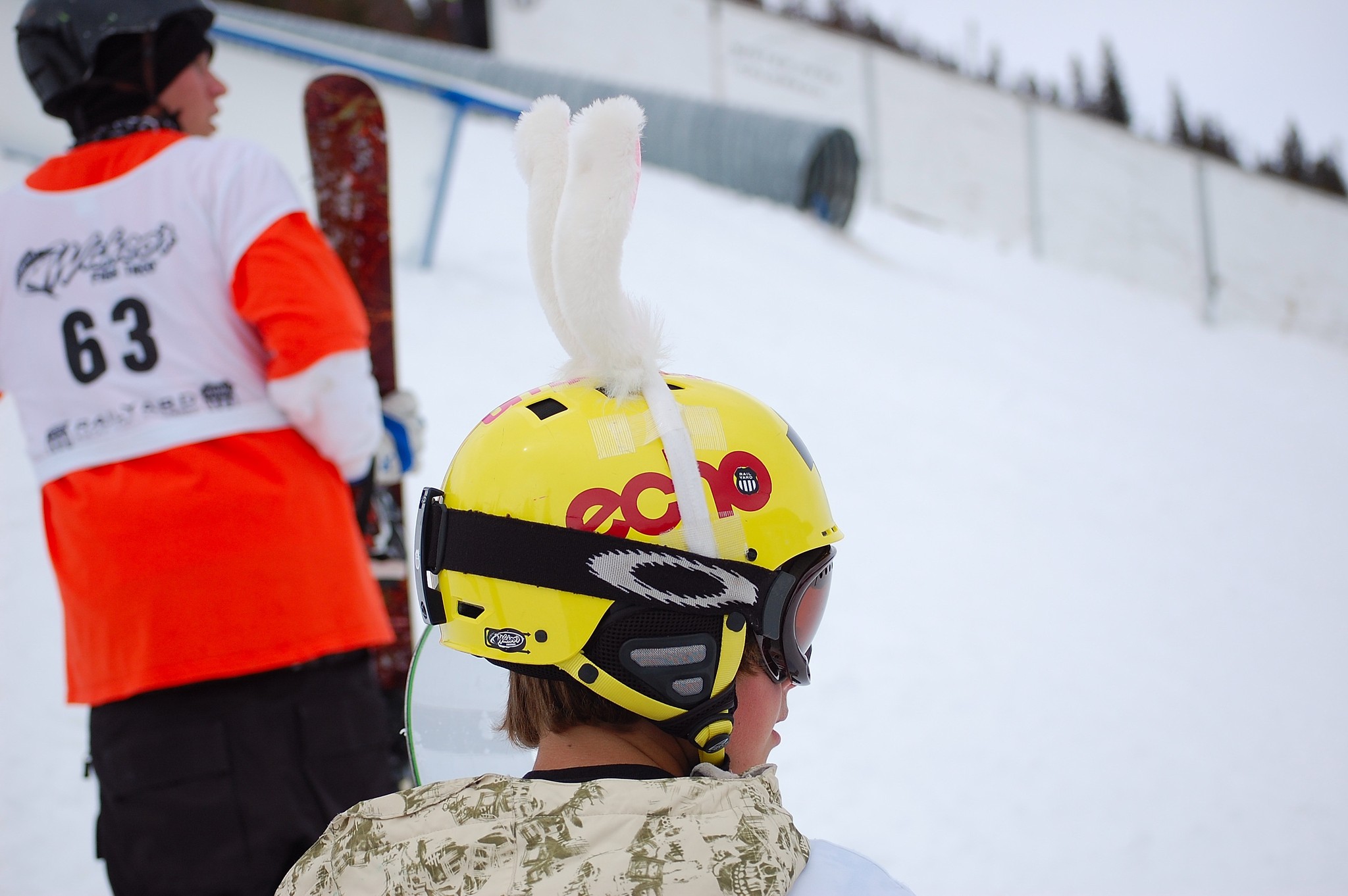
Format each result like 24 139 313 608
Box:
56 23 219 140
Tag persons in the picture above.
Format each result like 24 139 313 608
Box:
273 373 843 895
2 1 427 896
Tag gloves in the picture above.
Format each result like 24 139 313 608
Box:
381 394 429 490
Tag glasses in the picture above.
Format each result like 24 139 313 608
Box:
750 542 837 688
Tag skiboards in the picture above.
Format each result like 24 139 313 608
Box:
302 69 413 698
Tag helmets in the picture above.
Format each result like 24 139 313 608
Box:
435 370 837 677
16 0 212 117
